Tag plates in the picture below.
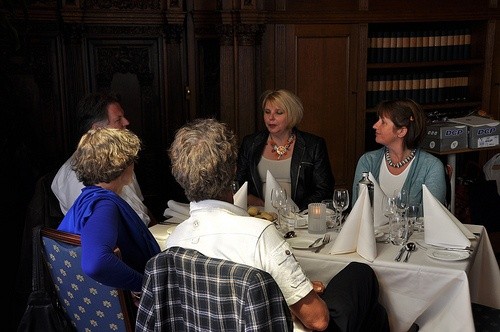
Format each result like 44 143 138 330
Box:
426 247 470 261
286 237 322 249
295 224 308 229
374 229 384 238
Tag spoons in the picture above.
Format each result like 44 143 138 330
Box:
403 242 415 262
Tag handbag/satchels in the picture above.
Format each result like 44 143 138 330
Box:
16 224 72 332
482 153 500 196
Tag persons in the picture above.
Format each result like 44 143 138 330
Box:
352 97 446 218
51 95 151 227
56 122 161 328
156 119 392 332
236 89 334 213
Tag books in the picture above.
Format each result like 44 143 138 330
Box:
367 29 479 106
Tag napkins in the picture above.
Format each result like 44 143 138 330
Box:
422 183 477 250
233 181 248 211
264 169 300 214
329 183 378 263
368 171 393 228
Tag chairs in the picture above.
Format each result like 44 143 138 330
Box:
37 173 65 229
144 247 278 332
39 228 136 332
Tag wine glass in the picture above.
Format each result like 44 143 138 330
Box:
271 188 287 231
333 189 349 233
382 189 409 230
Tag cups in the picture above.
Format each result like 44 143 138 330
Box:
389 215 408 245
322 200 337 231
231 180 239 195
280 207 295 233
409 202 422 232
308 203 327 234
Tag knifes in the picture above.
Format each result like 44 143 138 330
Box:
395 245 406 262
308 238 322 247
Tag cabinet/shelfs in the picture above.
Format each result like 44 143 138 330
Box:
365 59 485 111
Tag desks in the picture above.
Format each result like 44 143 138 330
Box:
422 145 500 217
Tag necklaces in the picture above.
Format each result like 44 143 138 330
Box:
269 132 295 158
385 146 417 168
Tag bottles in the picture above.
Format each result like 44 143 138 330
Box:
366 72 470 108
368 28 472 64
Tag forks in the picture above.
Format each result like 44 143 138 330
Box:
315 235 330 253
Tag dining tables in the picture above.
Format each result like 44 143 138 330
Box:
147 216 500 332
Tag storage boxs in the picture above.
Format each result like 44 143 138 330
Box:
421 122 467 153
448 116 500 148
